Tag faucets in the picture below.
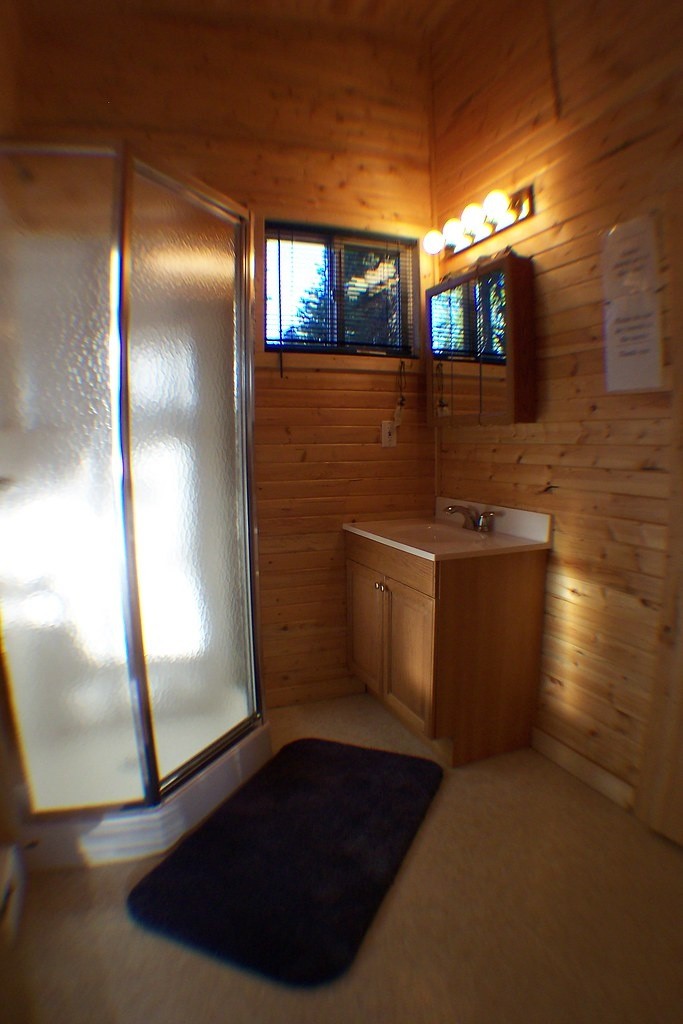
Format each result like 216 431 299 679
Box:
445 503 477 532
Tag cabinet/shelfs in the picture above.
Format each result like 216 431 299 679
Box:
341 518 552 768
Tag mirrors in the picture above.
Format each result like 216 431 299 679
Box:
425 255 535 426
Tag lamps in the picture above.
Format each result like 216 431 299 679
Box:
422 186 534 262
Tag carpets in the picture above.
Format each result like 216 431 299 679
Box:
125 738 447 993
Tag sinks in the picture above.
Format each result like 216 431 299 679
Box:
380 521 487 547
